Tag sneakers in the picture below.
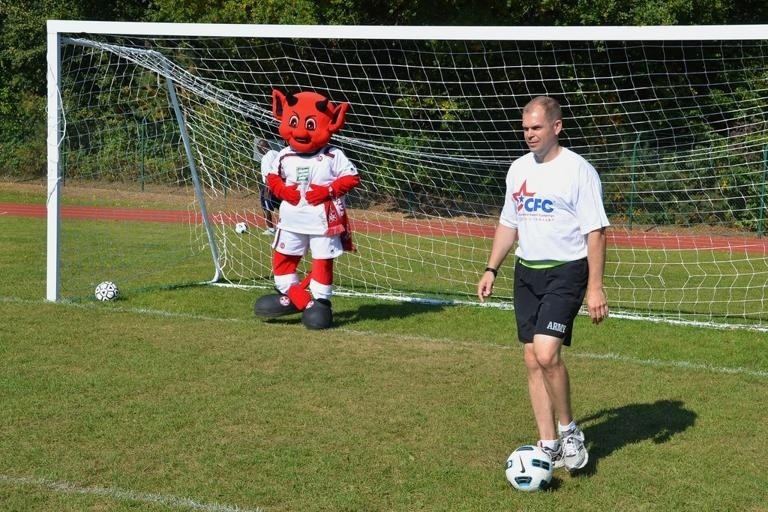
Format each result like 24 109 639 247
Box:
536 428 589 472
262 227 274 235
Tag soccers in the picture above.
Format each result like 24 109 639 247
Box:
95 281 119 303
505 444 553 493
235 222 248 234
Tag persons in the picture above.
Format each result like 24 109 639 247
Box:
475 95 612 473
254 139 287 237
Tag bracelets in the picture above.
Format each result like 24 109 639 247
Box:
484 267 498 279
